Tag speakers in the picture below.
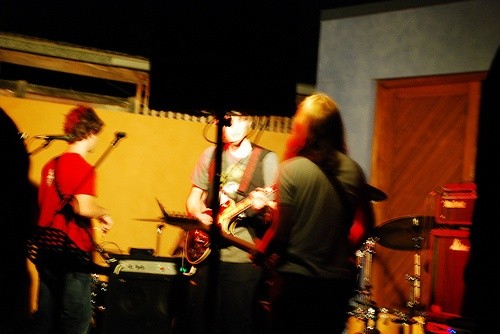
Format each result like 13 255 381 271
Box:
148 0 306 117
424 229 471 329
102 253 191 334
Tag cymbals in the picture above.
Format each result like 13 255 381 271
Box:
372 216 462 251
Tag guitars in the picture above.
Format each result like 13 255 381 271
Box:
185 180 297 265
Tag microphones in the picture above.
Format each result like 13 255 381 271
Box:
34 133 71 141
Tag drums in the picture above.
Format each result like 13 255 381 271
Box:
343 305 426 334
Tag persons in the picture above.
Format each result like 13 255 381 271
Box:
34 105 114 334
209 89 376 334
0 106 41 334
186 111 279 334
